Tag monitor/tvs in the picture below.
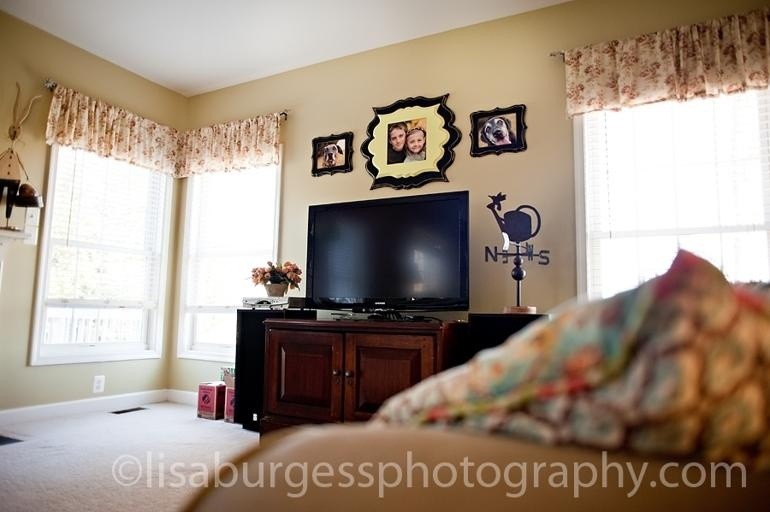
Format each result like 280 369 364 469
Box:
305 190 470 314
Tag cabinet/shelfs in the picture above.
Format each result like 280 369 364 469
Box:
259 316 468 439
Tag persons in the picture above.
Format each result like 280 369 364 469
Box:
387 123 409 164
403 129 427 163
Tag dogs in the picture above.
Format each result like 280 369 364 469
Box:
318 144 343 168
480 116 516 146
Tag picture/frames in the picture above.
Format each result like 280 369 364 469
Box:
310 92 528 190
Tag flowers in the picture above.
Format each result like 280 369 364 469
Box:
251 260 302 293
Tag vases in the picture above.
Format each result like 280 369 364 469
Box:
265 283 286 296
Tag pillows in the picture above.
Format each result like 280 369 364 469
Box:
369 250 769 473
